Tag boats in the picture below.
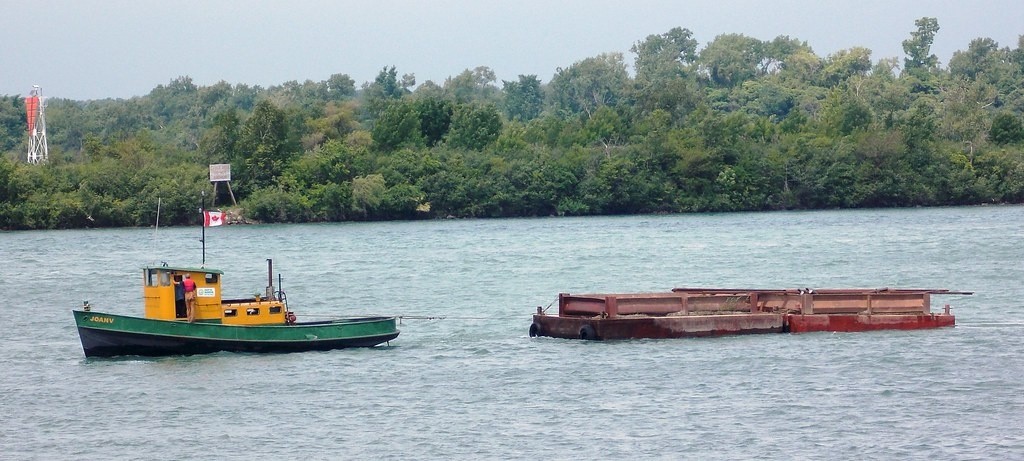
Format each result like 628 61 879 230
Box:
72 191 403 359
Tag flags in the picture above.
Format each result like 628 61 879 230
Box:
203 211 226 227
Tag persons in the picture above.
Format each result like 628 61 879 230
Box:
173 273 197 322
